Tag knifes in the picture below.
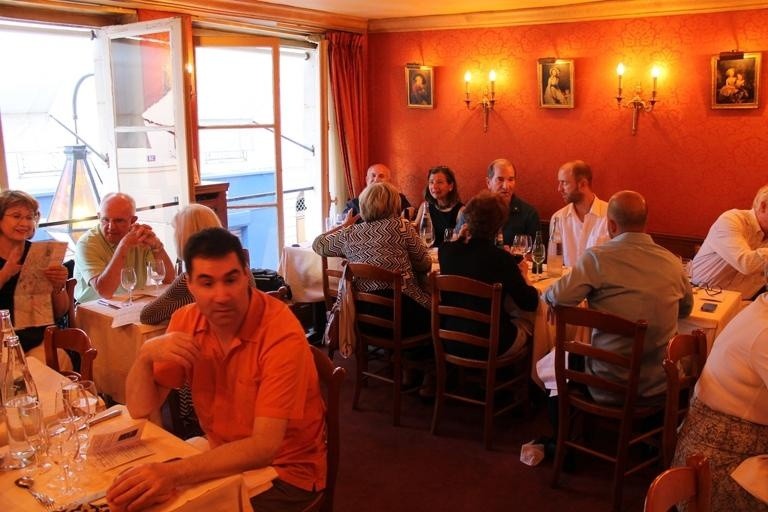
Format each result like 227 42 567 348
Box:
97 299 121 311
76 409 121 432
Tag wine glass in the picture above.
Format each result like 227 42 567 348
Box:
120 267 138 308
0 375 101 505
148 258 166 299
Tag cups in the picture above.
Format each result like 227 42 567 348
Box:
324 213 347 233
677 257 693 290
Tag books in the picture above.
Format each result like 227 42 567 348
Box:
12 240 69 331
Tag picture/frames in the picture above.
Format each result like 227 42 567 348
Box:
709 49 762 110
537 59 575 108
406 64 435 109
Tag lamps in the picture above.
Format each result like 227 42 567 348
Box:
463 66 497 133
613 61 662 135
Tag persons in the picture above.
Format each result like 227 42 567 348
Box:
307 156 768 511
719 67 739 103
1 189 74 375
734 72 752 99
410 73 431 105
104 226 329 512
543 67 568 105
138 204 257 435
72 191 176 308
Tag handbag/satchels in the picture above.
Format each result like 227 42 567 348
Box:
325 277 368 367
252 268 286 292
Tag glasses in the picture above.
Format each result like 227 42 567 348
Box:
690 282 721 296
6 212 37 220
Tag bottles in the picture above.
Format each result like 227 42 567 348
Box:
410 200 565 285
1 308 46 451
